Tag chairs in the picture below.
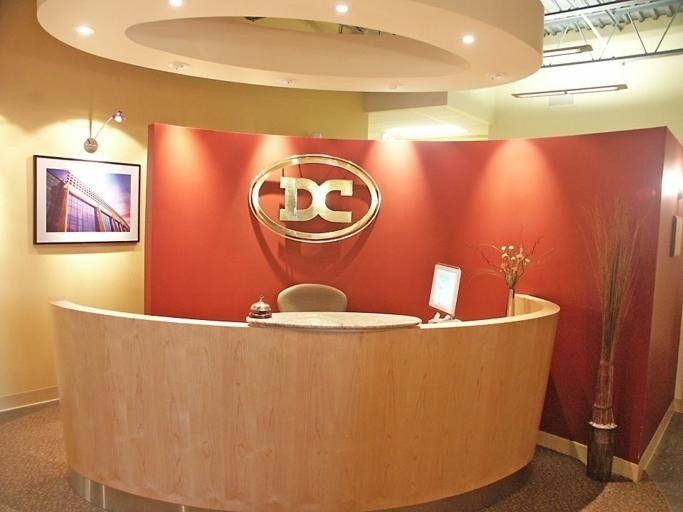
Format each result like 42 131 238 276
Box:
277 283 348 313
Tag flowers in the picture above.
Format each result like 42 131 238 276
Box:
480 236 543 290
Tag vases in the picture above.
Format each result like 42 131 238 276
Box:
582 420 618 481
507 287 516 316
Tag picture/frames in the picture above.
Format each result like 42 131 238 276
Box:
32 154 141 245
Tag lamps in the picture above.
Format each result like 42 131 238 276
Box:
83 111 123 154
